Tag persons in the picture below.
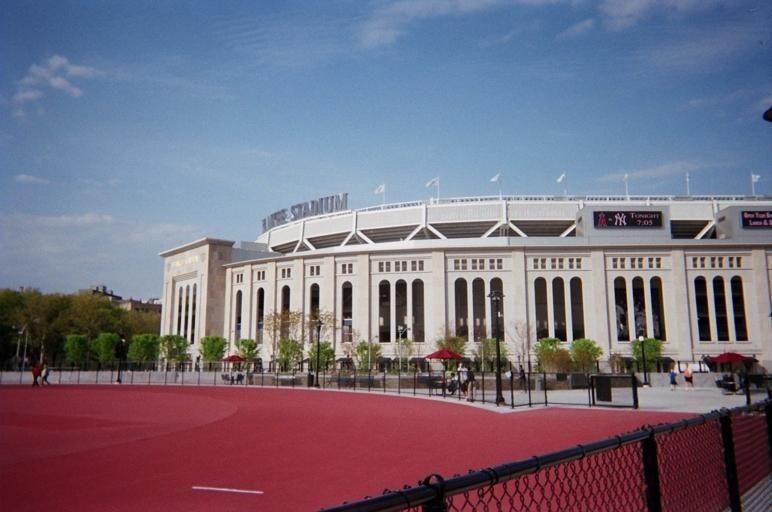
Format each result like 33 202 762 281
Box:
30 365 39 386
666 368 679 390
40 362 50 387
229 365 244 386
447 363 478 402
516 364 534 395
681 362 697 391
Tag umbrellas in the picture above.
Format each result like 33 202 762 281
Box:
221 356 247 363
709 353 757 365
426 349 463 361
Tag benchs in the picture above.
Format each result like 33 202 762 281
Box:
426 378 446 397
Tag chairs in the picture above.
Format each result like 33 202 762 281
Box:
326 374 375 391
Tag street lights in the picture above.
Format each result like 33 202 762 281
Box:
488 287 507 403
309 316 324 387
636 329 650 388
